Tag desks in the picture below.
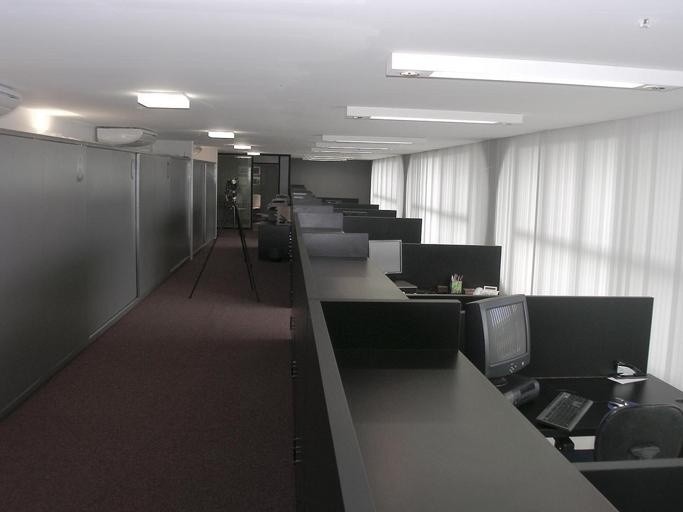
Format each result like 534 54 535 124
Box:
518 370 681 484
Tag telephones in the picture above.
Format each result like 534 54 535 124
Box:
473 286 500 296
616 360 646 376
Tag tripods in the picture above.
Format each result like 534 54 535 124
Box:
189 200 260 303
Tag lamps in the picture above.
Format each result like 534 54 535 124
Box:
322 135 424 145
345 106 523 125
207 131 262 158
137 92 191 109
302 142 390 159
386 52 682 93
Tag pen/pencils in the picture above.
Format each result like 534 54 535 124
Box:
449 272 464 280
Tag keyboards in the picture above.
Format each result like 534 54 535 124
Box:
536 391 595 432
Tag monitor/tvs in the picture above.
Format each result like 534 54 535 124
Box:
369 239 403 275
460 295 541 406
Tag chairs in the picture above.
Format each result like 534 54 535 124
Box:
591 401 680 473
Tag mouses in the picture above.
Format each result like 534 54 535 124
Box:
609 397 629 407
